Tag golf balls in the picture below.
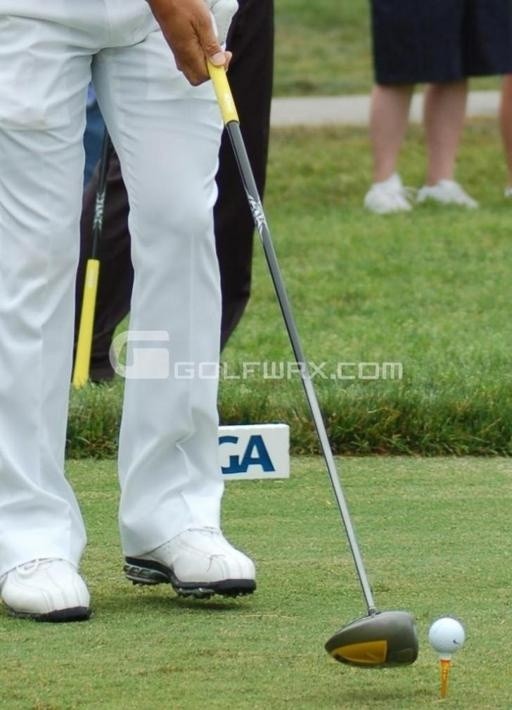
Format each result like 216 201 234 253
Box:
429 618 465 655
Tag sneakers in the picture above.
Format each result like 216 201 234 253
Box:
0 558 91 622
363 174 411 213
124 527 256 600
415 180 479 209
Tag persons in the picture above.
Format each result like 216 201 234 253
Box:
363 1 479 214
0 0 256 621
474 1 511 197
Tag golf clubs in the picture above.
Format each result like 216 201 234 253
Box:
205 50 421 667
